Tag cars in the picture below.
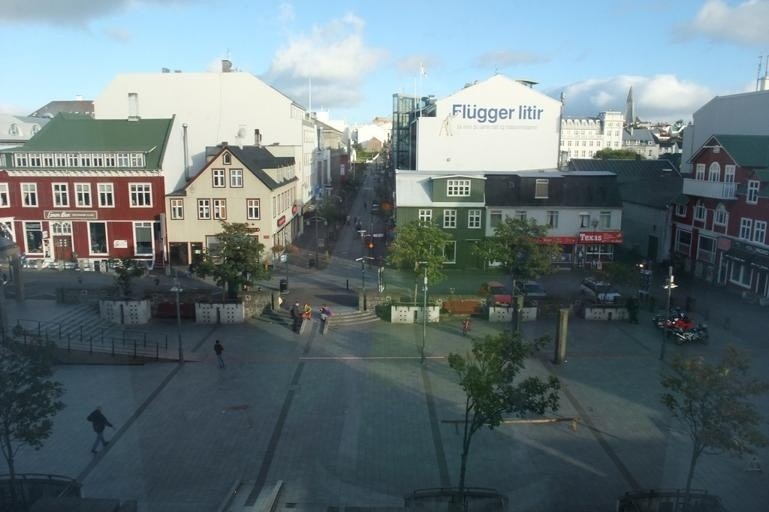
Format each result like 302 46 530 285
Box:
579 276 622 306
479 279 547 307
371 204 381 215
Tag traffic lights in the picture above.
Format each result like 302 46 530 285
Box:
368 243 374 248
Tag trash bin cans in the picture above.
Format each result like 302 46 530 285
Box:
280 279 288 292
309 258 315 266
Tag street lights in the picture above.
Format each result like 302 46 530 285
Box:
303 213 328 268
659 266 678 361
170 279 184 365
332 195 342 204
635 263 644 324
422 268 427 363
356 256 375 289
357 223 373 270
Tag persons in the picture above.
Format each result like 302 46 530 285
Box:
302 303 312 320
291 301 302 332
214 340 225 367
320 304 332 321
87 405 113 453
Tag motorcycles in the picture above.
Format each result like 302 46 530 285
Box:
652 306 708 345
